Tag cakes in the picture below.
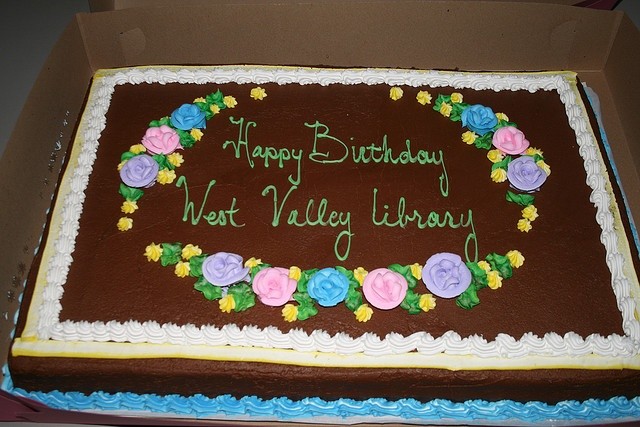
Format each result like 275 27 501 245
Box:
2 65 640 421
